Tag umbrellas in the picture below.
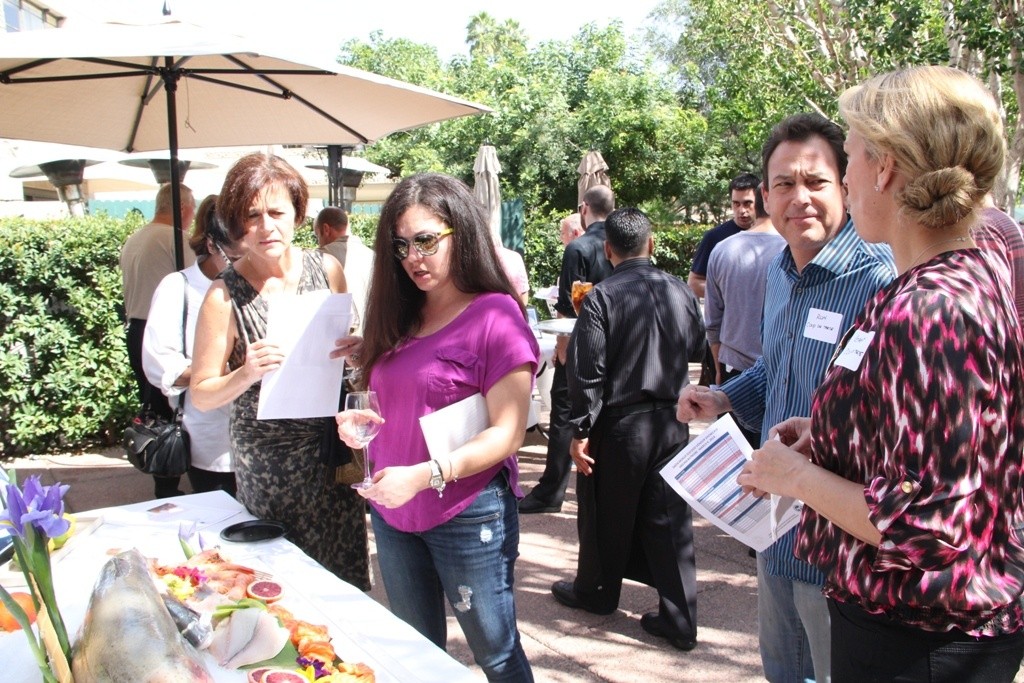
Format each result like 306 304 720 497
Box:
0 5 493 276
473 146 503 249
577 151 610 213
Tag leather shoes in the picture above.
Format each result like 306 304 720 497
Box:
552 580 619 615
641 612 698 650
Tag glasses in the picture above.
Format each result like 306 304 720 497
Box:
393 228 454 261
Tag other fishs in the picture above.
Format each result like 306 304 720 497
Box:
71 552 215 683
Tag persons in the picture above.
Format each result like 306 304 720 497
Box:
558 213 585 248
703 179 790 559
188 149 377 592
971 179 1024 336
490 239 531 311
315 205 380 395
676 114 901 682
515 186 615 513
120 183 196 500
738 67 1024 683
140 195 255 497
551 208 707 651
336 169 542 683
687 173 764 392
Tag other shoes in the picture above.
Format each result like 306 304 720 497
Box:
519 493 562 513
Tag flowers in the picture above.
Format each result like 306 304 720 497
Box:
0 472 77 683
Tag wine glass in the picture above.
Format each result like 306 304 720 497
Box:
342 391 386 490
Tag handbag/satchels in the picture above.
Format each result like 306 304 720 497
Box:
123 411 191 477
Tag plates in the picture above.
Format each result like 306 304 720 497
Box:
221 518 293 543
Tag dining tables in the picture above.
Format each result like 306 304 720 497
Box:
0 489 490 683
